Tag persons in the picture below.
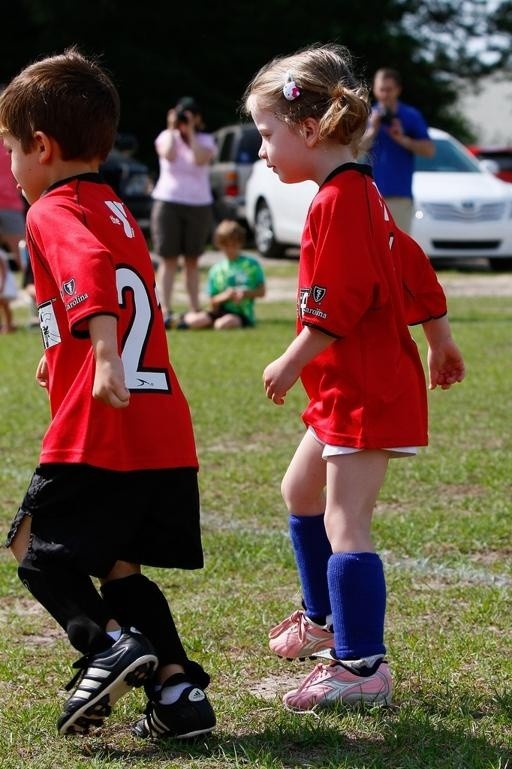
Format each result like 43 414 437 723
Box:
244 48 466 714
363 66 436 234
0 127 41 334
152 96 265 332
0 49 217 743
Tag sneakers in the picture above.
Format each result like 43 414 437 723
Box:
131 682 217 738
57 627 158 735
268 611 335 660
283 660 394 709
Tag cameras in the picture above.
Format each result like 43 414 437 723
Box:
177 106 186 125
379 106 396 126
